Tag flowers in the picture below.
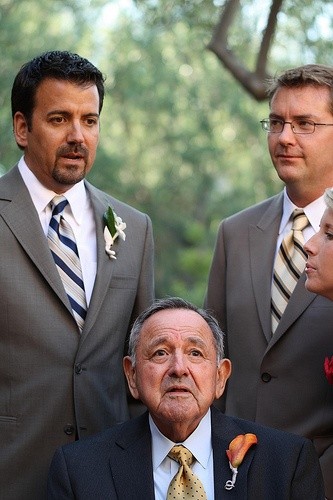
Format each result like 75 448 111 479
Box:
224 433 257 491
102 206 126 260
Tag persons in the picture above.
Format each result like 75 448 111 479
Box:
0 50 155 500
47 297 325 500
203 64 333 500
303 188 333 387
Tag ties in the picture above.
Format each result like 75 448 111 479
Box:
45 195 89 337
271 208 309 334
165 444 208 500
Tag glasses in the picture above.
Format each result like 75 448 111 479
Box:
260 114 333 135
